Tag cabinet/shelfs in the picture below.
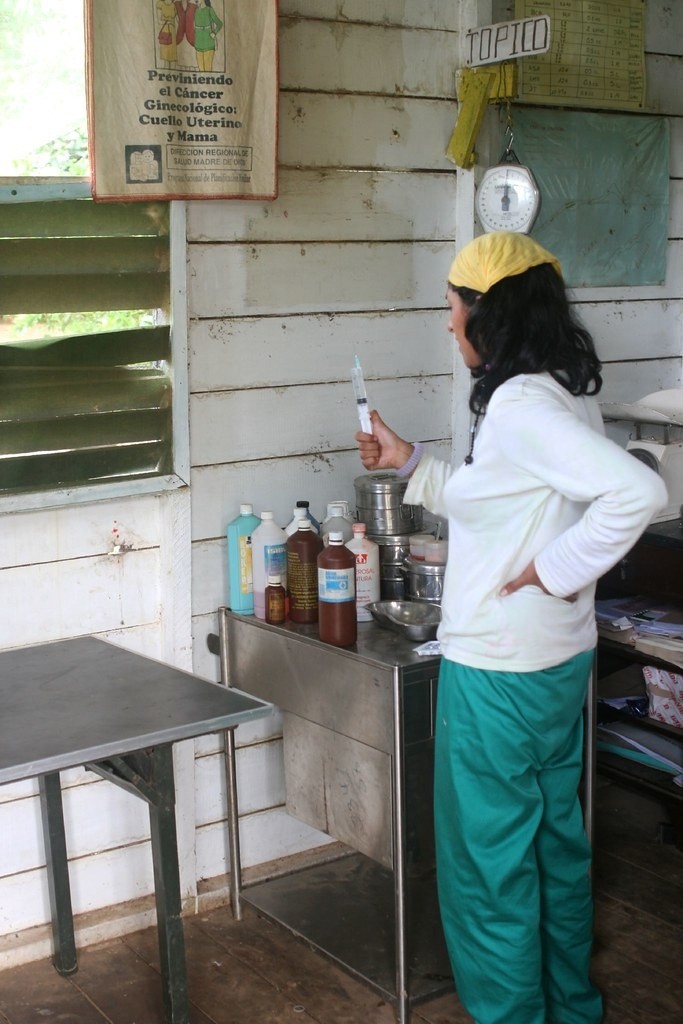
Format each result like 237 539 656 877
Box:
594 516 683 803
217 606 593 1023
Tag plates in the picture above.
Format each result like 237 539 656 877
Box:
363 599 443 642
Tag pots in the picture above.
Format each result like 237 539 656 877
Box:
351 471 449 609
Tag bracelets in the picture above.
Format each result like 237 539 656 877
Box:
395 442 423 479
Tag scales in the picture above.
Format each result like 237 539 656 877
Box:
474 127 543 235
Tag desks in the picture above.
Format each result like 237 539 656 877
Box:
0 634 274 1024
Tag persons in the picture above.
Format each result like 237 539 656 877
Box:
354 232 667 1024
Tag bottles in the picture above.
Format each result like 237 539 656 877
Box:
265 576 286 626
319 507 355 548
344 523 379 622
316 531 357 647
286 520 323 624
251 511 290 621
286 501 320 534
284 508 319 537
226 504 262 615
